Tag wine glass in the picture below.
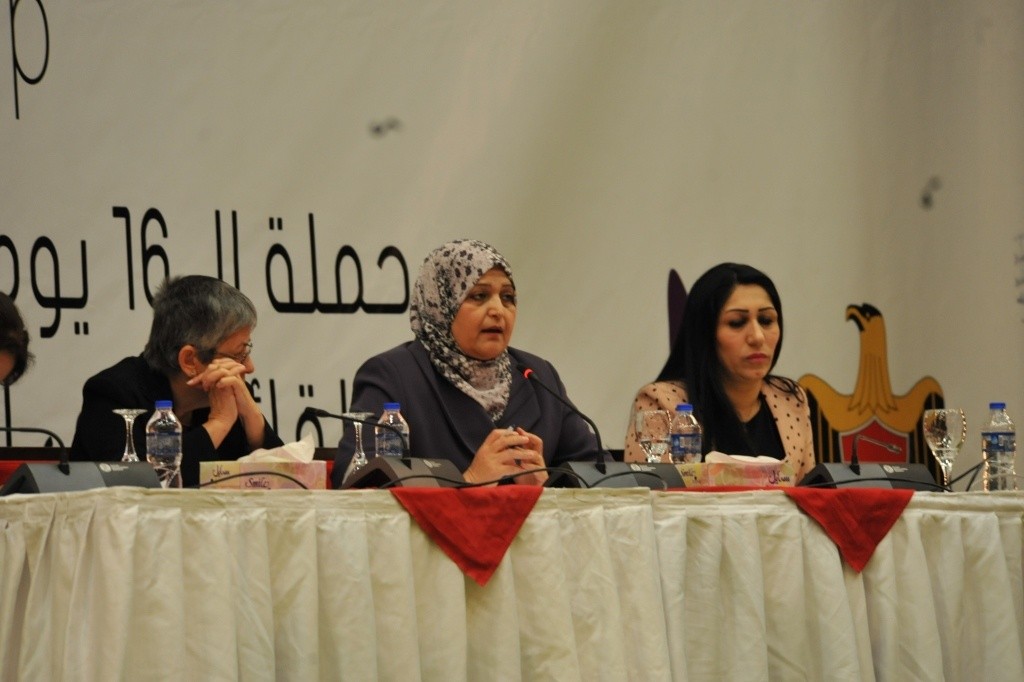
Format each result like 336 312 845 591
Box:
633 406 672 464
923 408 965 493
113 406 148 463
341 409 375 485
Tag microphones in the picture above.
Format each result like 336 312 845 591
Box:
518 362 607 474
306 405 410 457
849 433 904 477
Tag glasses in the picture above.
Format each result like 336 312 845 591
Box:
204 343 252 365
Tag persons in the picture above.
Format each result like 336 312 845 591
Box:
66 273 286 491
0 290 38 390
326 237 616 490
622 261 818 486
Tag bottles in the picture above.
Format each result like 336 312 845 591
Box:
669 401 702 465
147 401 184 489
376 403 412 463
981 400 1019 494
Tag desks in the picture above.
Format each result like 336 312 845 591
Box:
0 482 1024 680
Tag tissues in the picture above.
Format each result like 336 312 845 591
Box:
669 450 797 492
199 431 333 496
